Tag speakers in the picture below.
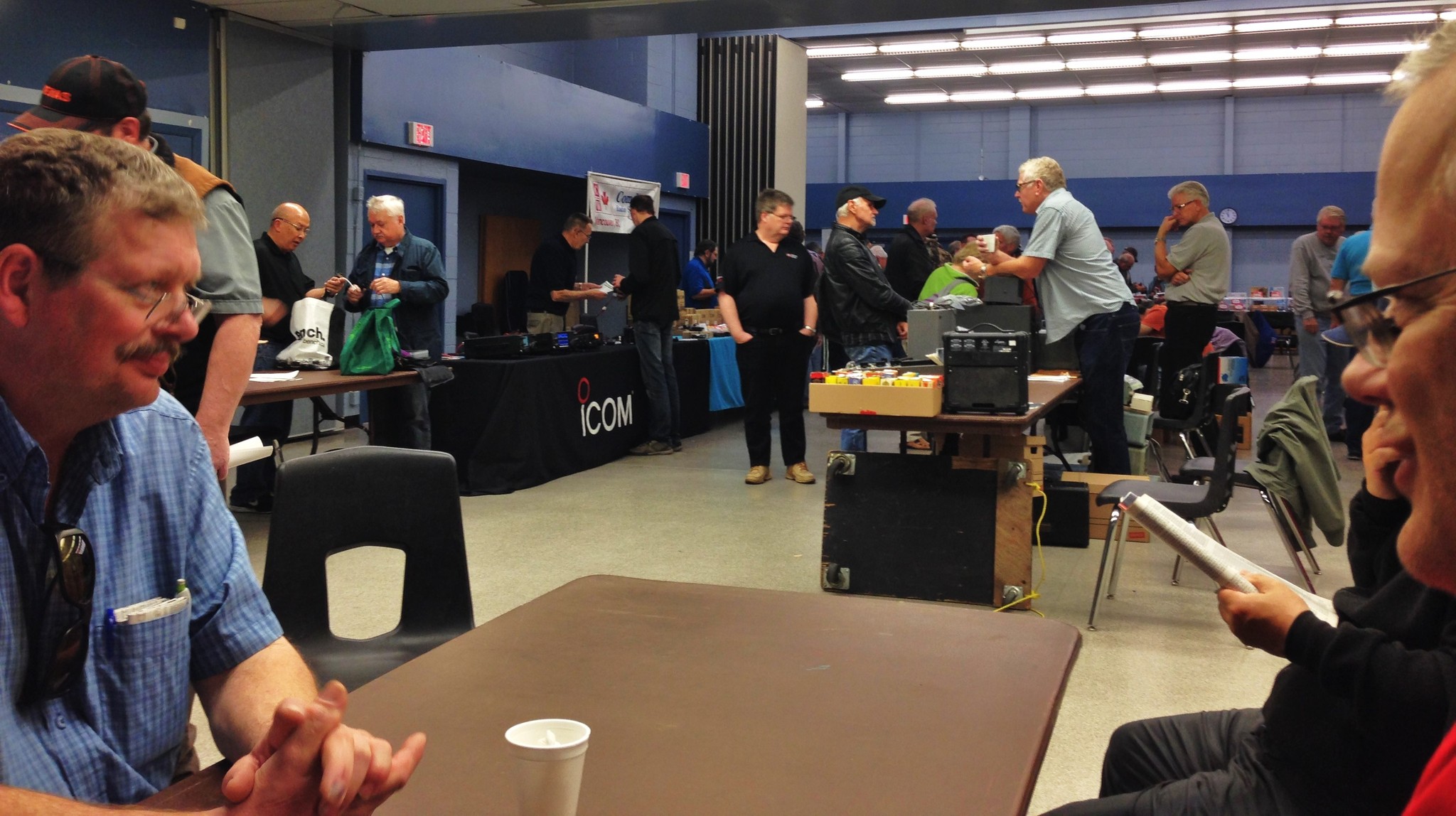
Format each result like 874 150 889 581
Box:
944 331 1033 414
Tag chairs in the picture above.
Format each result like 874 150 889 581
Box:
1088 414 1254 650
456 270 530 335
264 445 474 695
1170 375 1324 595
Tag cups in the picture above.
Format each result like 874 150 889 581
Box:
504 719 591 816
980 234 996 253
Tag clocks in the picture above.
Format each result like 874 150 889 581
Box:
1220 207 1238 224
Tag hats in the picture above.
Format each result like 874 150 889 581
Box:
835 185 887 210
1124 246 1138 262
7 55 148 133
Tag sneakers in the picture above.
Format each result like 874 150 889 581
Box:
745 466 772 483
785 460 815 483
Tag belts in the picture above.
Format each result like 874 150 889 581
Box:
751 327 798 336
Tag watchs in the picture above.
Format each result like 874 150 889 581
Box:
1153 237 1166 246
324 288 340 299
977 263 988 280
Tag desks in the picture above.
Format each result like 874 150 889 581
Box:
1216 309 1297 366
369 331 743 495
240 367 420 455
343 573 1083 816
820 369 1083 475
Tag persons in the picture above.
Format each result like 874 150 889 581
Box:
885 197 938 451
226 201 347 513
680 240 719 310
788 219 1042 322
611 195 687 456
5 55 264 485
526 212 608 335
0 127 427 816
1038 401 1456 816
1102 234 1168 294
815 186 913 452
343 193 449 452
1330 17 1456 816
714 189 820 485
1287 205 1400 460
962 155 1138 475
1153 178 1232 457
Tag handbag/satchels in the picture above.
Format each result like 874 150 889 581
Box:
1158 367 1201 420
912 279 973 308
338 299 401 376
274 296 335 369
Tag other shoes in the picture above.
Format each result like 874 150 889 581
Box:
627 440 673 455
1334 428 1346 442
672 440 684 451
1347 444 1362 460
229 485 273 514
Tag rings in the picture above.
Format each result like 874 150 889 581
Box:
614 278 615 281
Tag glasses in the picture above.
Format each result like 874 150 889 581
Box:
1169 200 1196 214
574 229 592 240
1331 268 1455 368
766 211 797 220
271 217 311 235
1016 180 1037 189
63 263 211 328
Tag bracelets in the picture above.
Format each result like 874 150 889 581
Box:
578 282 582 291
805 326 817 336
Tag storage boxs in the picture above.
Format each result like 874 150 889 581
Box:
627 288 722 328
1216 412 1254 449
808 379 944 417
934 434 1045 496
1063 471 1150 543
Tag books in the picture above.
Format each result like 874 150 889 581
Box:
1114 492 1339 629
